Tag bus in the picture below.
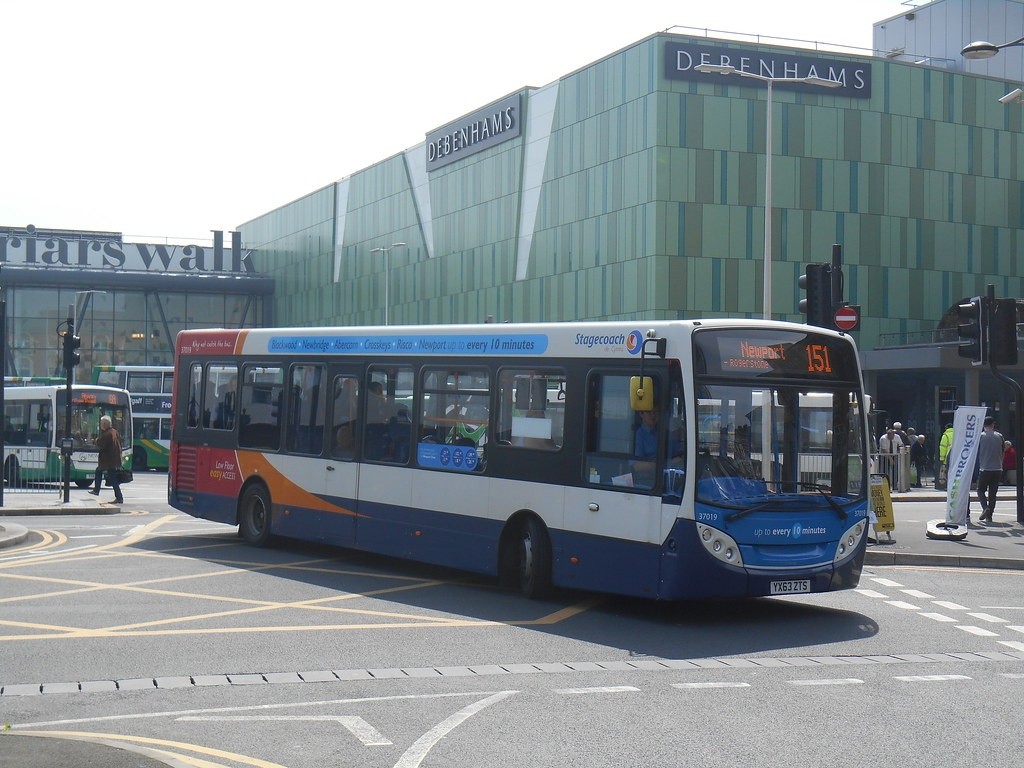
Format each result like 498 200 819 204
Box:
3 377 134 490
90 366 283 473
167 318 868 604
434 384 872 494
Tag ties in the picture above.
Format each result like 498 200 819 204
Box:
889 441 893 458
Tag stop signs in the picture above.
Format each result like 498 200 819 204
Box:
835 308 857 329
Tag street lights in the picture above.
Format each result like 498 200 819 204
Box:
693 63 844 492
369 242 406 325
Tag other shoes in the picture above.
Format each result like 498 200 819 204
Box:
911 483 921 488
108 499 123 504
979 507 991 520
88 490 99 495
986 517 992 522
966 517 970 523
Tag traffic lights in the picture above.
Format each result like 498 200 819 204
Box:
797 263 832 329
70 334 81 366
958 297 983 365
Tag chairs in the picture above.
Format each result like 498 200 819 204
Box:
188 417 475 467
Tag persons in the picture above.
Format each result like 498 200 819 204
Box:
825 422 927 489
87 416 124 504
210 373 412 463
976 416 1005 525
626 399 685 482
946 449 971 524
939 423 955 490
998 441 1017 486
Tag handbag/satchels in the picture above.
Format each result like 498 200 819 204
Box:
104 466 133 486
910 464 917 485
